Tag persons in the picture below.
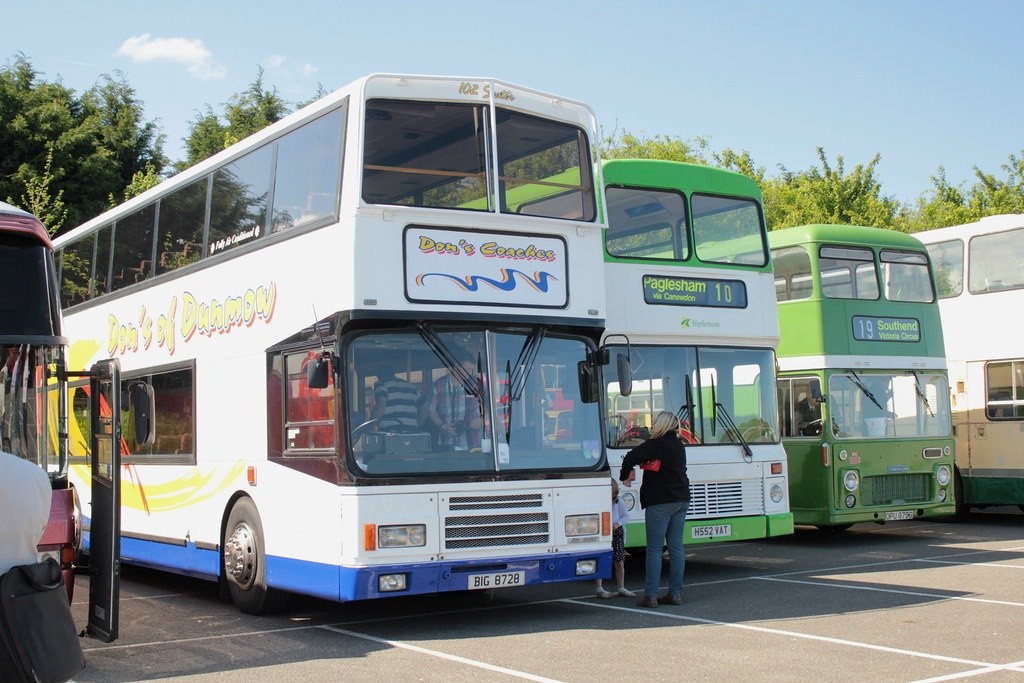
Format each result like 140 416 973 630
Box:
371 351 485 452
792 391 839 437
0 451 53 577
620 411 691 608
594 478 636 597
862 375 897 438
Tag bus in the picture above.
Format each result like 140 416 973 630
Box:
606 223 958 533
445 157 798 555
767 212 1024 524
0 198 157 613
29 71 637 615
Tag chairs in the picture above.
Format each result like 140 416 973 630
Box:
157 434 180 454
134 260 160 283
160 251 182 274
178 243 202 267
122 268 140 288
176 433 193 454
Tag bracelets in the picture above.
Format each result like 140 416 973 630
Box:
440 422 445 430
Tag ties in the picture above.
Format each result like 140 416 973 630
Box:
811 408 813 411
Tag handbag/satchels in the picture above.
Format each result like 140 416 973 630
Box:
0 557 85 683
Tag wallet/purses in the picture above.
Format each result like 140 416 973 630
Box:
640 459 661 471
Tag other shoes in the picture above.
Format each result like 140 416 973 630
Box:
636 596 657 607
657 594 681 605
597 590 614 599
618 588 636 597
